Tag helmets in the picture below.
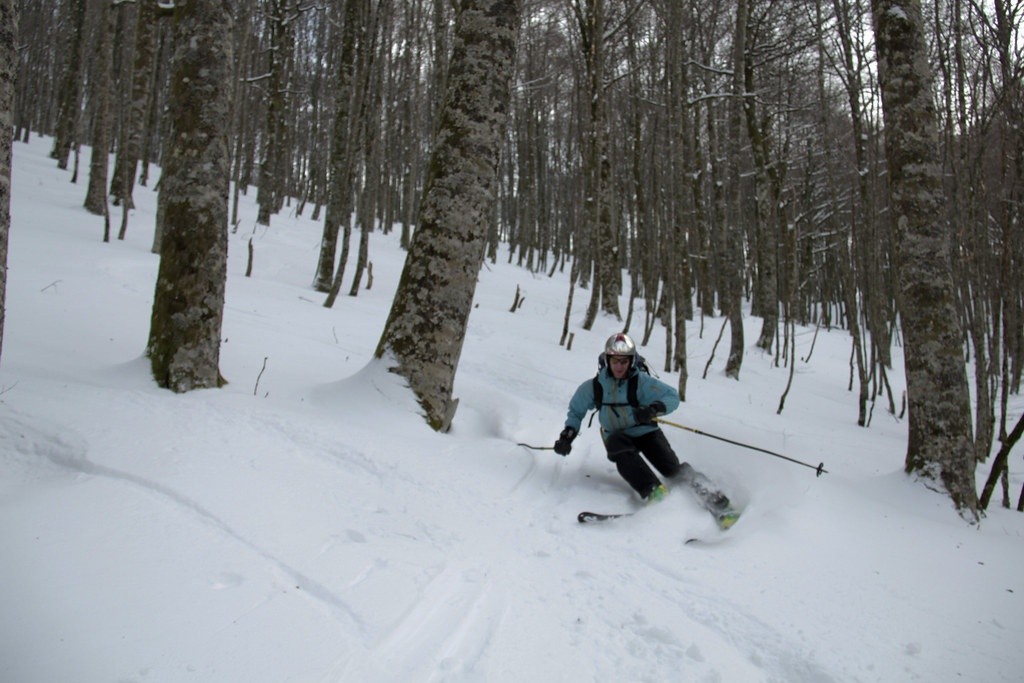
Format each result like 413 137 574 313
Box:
606 333 636 356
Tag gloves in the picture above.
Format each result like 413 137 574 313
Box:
554 429 577 454
638 407 655 423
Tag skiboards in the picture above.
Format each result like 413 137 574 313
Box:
577 461 751 545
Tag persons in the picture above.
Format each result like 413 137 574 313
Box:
553 333 742 530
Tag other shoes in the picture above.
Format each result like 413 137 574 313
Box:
646 487 666 502
